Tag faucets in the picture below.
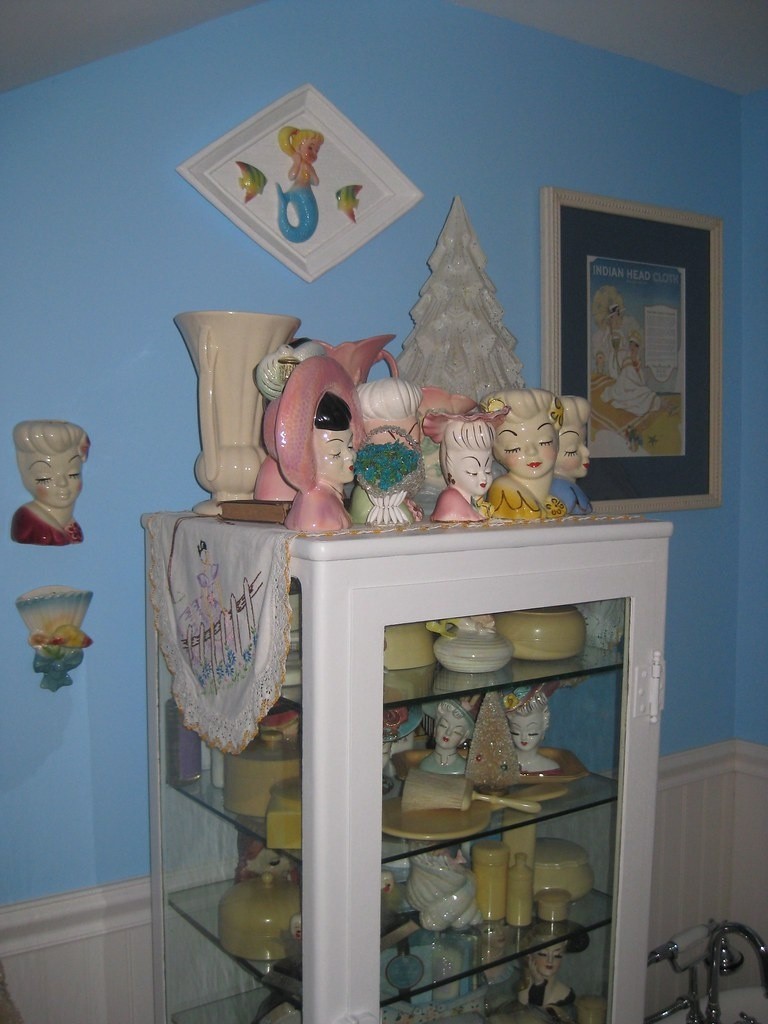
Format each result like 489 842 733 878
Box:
707 921 768 1021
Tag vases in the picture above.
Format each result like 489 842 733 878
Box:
173 309 302 521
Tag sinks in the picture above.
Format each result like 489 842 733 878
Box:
658 984 768 1024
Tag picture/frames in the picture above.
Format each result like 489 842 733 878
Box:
540 185 723 514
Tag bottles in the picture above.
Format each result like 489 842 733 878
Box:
507 854 534 927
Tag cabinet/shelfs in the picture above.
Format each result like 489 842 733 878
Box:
140 511 675 1024
